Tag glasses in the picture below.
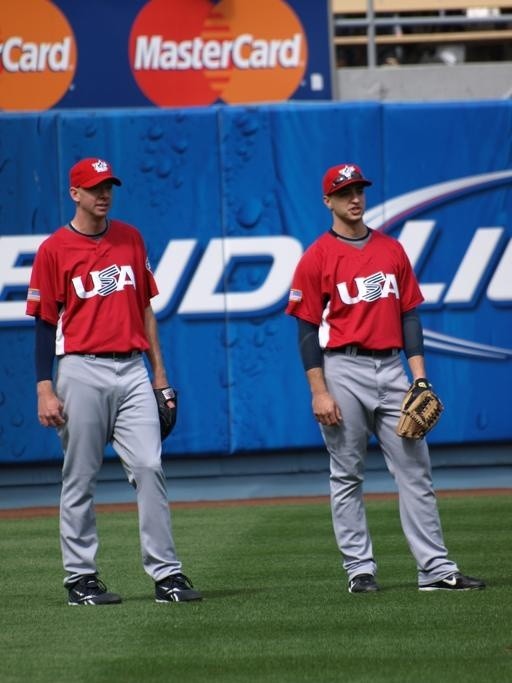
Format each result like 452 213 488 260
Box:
326 171 362 196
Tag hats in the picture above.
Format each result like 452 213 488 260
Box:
70 158 122 190
323 163 372 197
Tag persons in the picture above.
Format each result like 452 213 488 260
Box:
283 162 486 595
26 159 204 605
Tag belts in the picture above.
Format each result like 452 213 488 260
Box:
330 346 401 356
72 348 143 358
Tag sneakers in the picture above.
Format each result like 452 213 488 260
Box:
67 575 122 606
347 574 381 593
155 573 203 602
418 572 487 592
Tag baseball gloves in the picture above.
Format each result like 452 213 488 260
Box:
396 378 443 440
153 386 176 440
